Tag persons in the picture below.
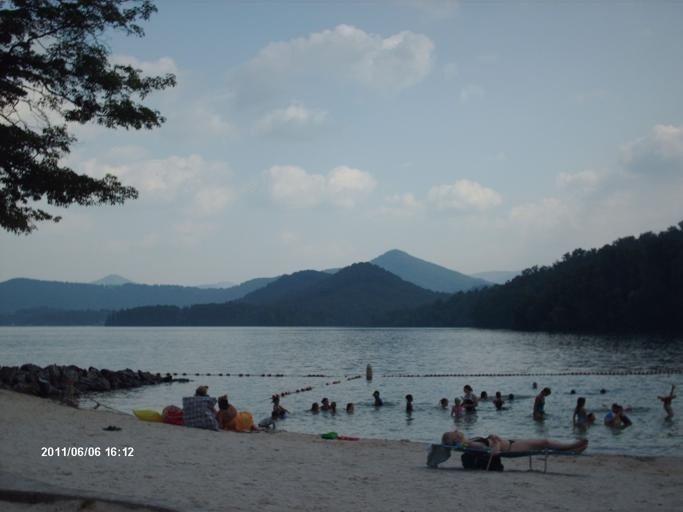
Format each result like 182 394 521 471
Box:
656 384 677 420
439 381 633 429
176 385 218 415
404 393 415 413
440 430 587 454
372 390 383 407
215 395 263 433
254 396 354 431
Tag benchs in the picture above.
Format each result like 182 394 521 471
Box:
431 439 591 472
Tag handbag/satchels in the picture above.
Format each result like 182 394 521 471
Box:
225 411 254 433
182 396 219 431
160 405 183 425
461 453 504 472
